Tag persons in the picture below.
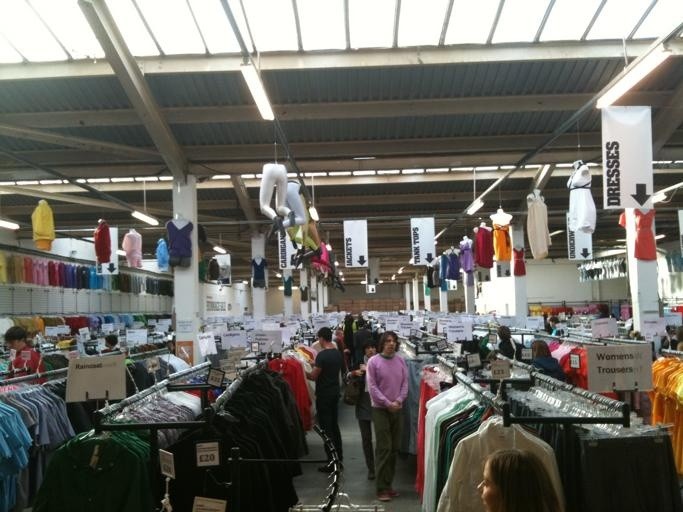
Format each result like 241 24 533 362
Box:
31 200 56 251
258 163 297 239
101 334 119 354
367 330 409 502
155 238 170 273
497 326 522 361
164 213 194 267
342 313 372 372
304 326 346 475
248 180 336 301
576 257 627 284
121 228 144 269
477 446 560 511
344 340 383 480
425 188 553 288
549 316 564 338
565 160 597 234
529 340 559 379
93 218 112 264
3 325 47 385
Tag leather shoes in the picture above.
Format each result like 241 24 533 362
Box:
367 472 374 479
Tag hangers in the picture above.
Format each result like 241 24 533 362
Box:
419 346 680 439
80 354 290 464
288 455 385 511
0 371 69 406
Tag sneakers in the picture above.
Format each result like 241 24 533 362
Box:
387 488 399 497
377 493 390 501
318 466 333 473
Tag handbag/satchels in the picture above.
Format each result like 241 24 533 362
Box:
343 364 360 405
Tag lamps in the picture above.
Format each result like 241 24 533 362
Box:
636 189 671 205
237 44 279 124
595 34 676 115
